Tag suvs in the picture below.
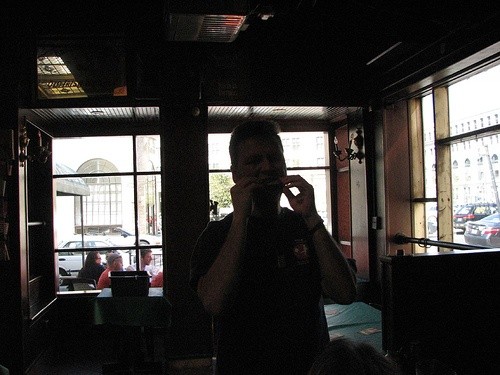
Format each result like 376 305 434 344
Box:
427 204 437 224
453 202 498 230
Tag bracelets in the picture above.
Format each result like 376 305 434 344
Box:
308 220 323 236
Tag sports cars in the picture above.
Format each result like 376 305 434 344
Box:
89 226 162 254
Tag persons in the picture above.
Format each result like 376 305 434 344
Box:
308 337 402 375
191 121 356 375
77 248 163 290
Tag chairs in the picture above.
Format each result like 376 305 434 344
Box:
110 271 149 298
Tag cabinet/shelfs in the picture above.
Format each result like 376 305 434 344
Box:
376 249 500 355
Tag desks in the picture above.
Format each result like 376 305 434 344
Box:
47 297 170 356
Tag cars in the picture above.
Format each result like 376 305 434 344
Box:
464 213 500 249
56 235 137 287
